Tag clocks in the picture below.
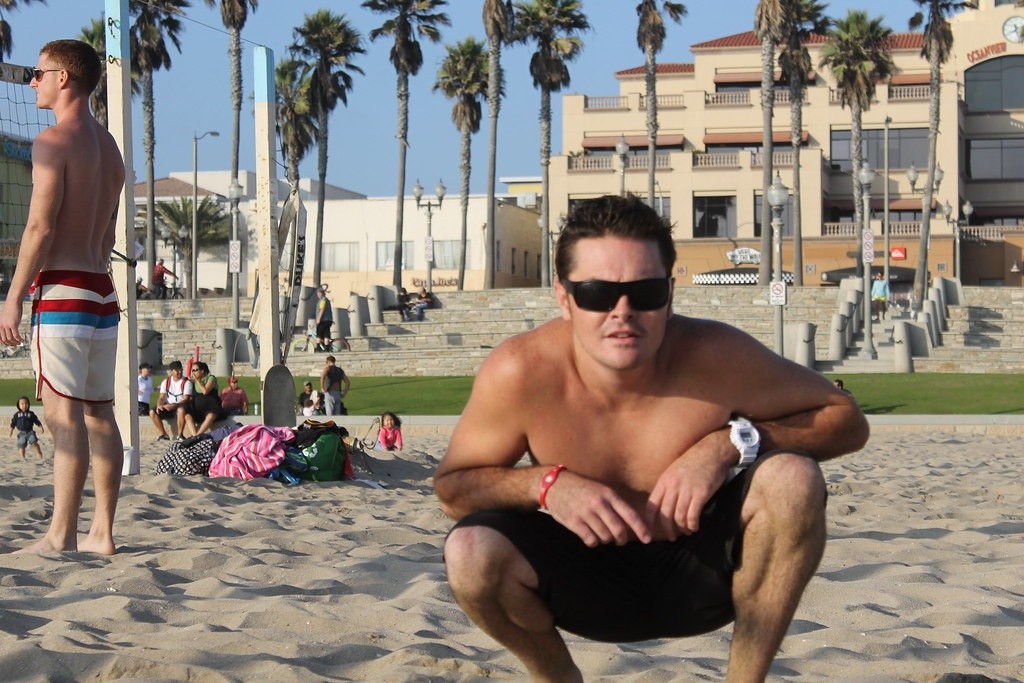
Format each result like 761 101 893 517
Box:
1002 16 1024 43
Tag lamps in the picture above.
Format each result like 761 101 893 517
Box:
1011 262 1020 272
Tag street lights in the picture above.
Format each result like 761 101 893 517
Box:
225 177 245 330
766 169 790 358
616 133 631 200
943 197 974 284
411 177 448 292
856 160 878 361
908 162 944 301
192 131 220 300
537 211 566 288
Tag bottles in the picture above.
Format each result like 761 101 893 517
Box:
254 403 258 415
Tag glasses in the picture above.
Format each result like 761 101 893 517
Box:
33 68 74 82
192 368 202 372
559 275 672 313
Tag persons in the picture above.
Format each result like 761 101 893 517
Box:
397 288 411 321
184 361 223 437
0 40 126 553
150 360 193 441
315 288 333 351
220 377 248 415
321 356 350 415
375 411 401 451
137 363 161 415
297 381 345 417
136 276 151 299
9 396 44 459
415 288 433 321
152 259 179 300
433 192 869 682
871 271 890 320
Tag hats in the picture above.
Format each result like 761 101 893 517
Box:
228 376 238 382
169 361 181 369
139 363 152 370
304 381 311 386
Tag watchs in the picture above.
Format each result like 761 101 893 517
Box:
727 415 760 467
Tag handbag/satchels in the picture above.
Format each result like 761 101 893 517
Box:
285 429 345 482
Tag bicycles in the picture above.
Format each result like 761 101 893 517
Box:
292 323 351 353
171 278 185 299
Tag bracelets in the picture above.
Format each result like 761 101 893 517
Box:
539 463 568 510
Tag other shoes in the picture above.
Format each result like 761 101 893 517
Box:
158 434 171 441
177 433 186 440
